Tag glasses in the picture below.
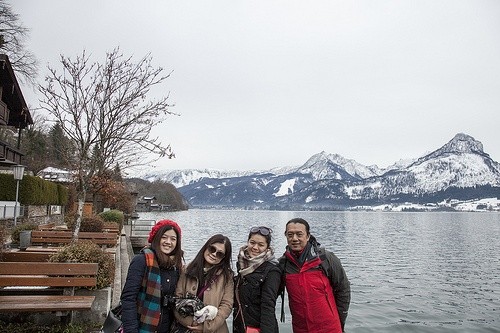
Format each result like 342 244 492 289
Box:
250 227 269 235
208 245 225 258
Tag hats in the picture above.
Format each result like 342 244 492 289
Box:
148 220 181 243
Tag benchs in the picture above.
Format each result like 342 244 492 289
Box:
0 221 119 333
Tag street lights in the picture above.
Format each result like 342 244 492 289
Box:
11 162 26 227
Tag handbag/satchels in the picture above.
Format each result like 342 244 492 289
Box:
102 310 124 333
245 325 261 333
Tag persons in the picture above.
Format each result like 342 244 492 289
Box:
120 219 183 333
233 226 280 333
278 218 351 333
176 234 234 333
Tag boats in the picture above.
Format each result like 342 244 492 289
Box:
130 220 156 249
